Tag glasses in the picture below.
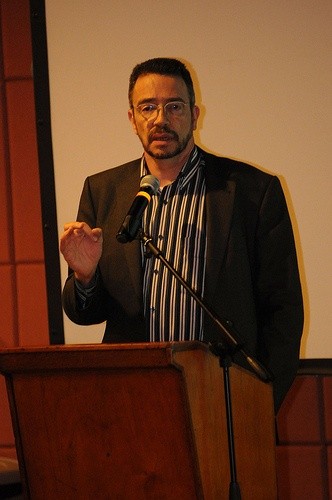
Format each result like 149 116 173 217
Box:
132 101 190 118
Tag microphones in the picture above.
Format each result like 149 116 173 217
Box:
116 174 160 244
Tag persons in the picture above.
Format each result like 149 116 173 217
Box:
62 55 305 450
